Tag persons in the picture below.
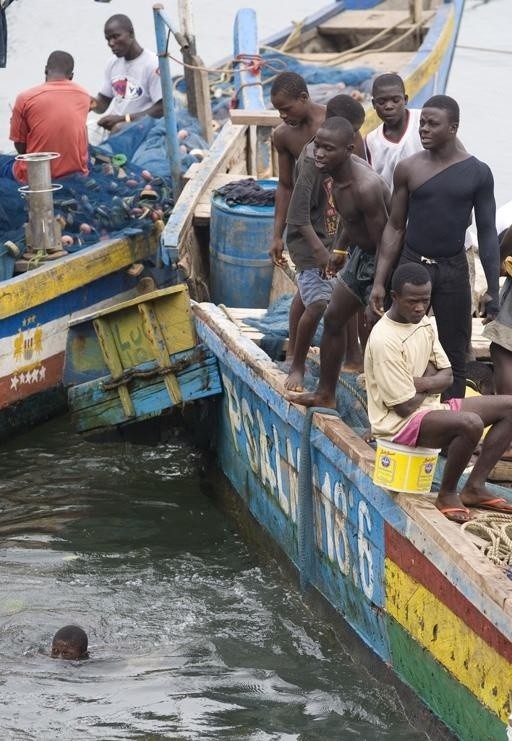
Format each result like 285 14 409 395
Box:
268 68 512 523
88 15 163 131
7 50 94 179
47 625 90 663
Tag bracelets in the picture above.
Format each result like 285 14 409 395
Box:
123 114 131 122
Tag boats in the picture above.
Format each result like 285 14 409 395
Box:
186 278 508 687
0 0 464 372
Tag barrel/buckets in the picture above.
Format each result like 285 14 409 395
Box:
372 437 445 495
209 176 291 307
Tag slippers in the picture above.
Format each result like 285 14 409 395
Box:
463 498 512 513
440 506 470 522
